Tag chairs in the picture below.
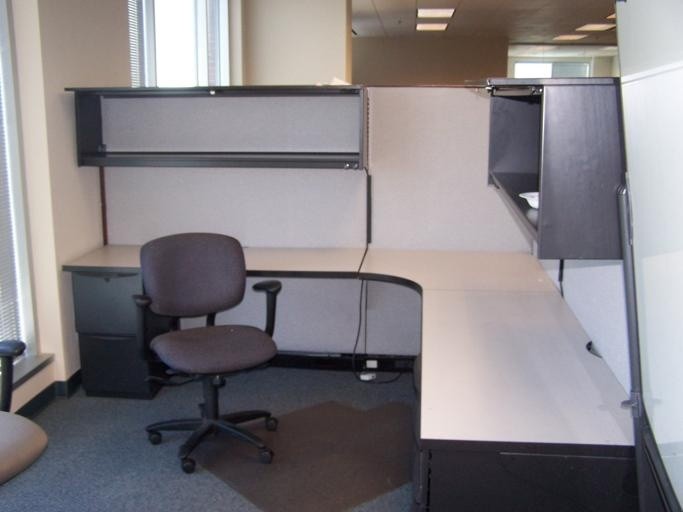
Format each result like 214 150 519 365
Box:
0 340 49 486
132 232 282 473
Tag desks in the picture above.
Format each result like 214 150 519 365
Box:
62 244 369 397
359 245 635 512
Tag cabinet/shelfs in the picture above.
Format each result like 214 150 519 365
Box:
63 86 369 170
487 77 626 260
